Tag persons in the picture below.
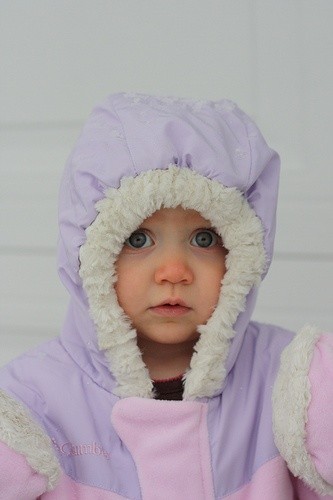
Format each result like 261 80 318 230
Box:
0 92 333 500
